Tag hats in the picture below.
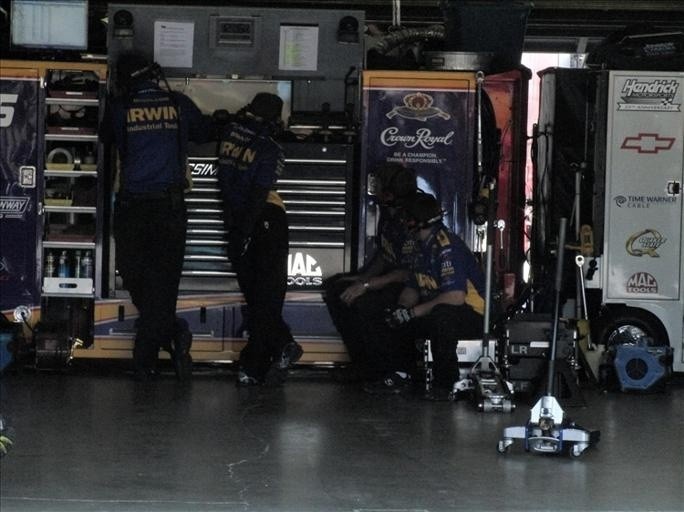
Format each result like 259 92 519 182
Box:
116 52 153 86
247 92 283 120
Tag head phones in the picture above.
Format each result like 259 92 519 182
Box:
403 186 427 228
375 163 397 207
114 52 162 93
246 92 284 128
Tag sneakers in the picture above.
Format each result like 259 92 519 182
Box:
131 369 166 380
363 374 413 396
238 361 263 387
422 388 453 401
277 341 304 370
172 327 193 386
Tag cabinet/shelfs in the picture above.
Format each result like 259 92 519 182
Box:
6 58 106 360
357 72 476 273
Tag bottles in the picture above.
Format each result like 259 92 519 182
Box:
44 251 93 290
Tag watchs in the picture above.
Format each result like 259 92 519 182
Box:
360 278 371 292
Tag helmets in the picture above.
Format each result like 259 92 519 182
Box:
380 178 443 231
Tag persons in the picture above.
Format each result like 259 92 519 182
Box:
326 168 425 383
371 192 485 393
216 91 301 384
100 54 228 384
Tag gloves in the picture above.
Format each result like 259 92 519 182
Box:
375 306 411 330
